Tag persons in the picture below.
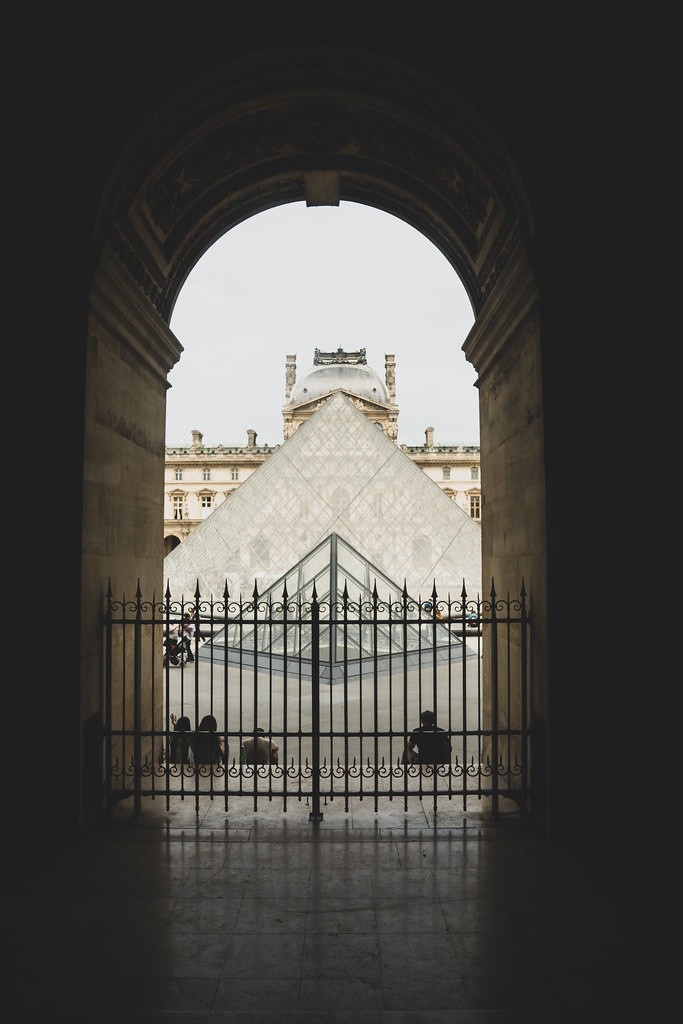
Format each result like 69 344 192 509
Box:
239 728 279 765
400 710 452 764
169 713 191 764
186 713 230 764
163 613 195 665
